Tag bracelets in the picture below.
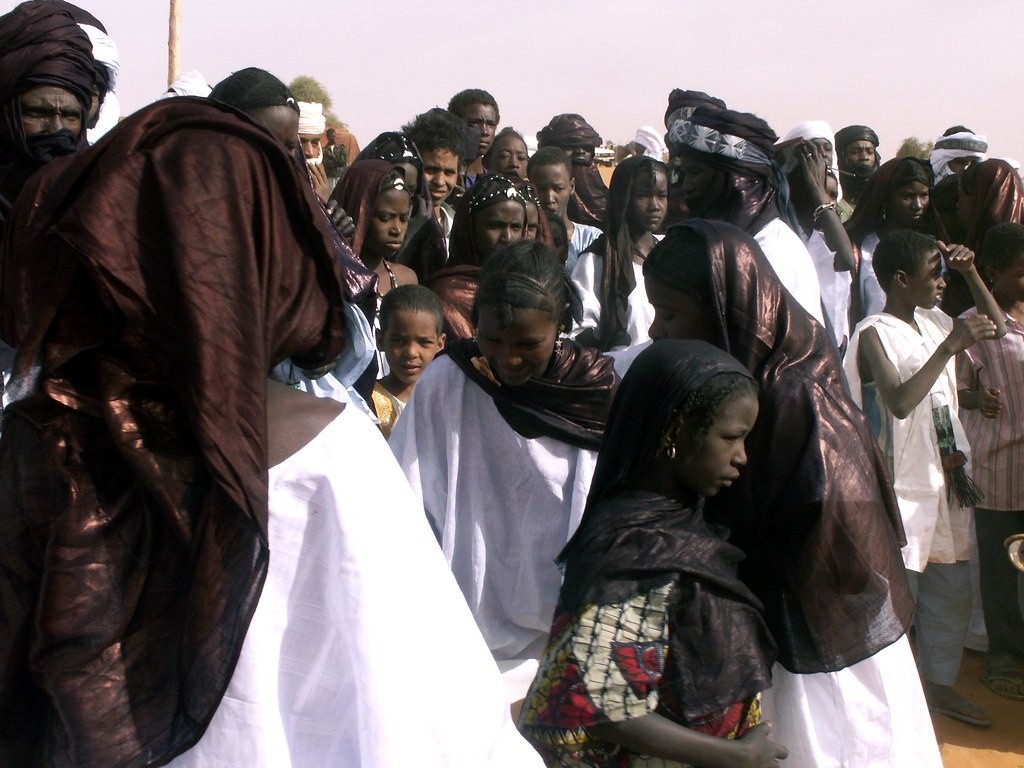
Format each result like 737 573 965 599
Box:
813 201 839 226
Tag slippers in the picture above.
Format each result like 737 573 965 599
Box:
984 674 1024 700
928 697 993 726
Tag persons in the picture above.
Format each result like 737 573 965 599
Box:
370 282 448 439
513 336 788 767
0 0 1023 768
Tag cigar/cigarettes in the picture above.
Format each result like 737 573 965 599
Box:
830 166 856 177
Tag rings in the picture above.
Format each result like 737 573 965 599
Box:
807 153 812 157
